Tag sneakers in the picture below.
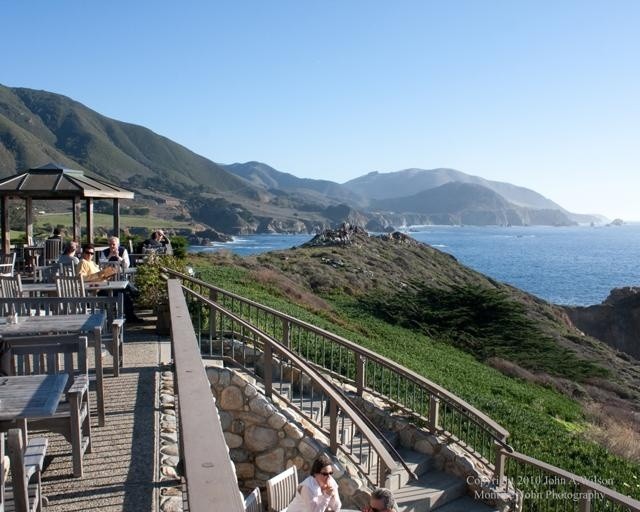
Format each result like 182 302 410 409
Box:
126 319 143 324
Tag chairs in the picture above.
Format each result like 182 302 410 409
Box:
0 426 49 512
1 235 168 377
244 464 361 512
0 335 92 479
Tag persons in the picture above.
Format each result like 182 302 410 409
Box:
137 232 164 254
75 244 144 324
45 227 62 266
154 230 170 246
100 236 130 282
56 241 80 266
285 455 342 512
362 487 400 512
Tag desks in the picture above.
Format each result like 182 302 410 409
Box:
1 312 107 427
1 374 74 451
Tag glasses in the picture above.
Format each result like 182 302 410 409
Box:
89 284 104 287
86 251 95 255
319 471 333 477
371 506 386 512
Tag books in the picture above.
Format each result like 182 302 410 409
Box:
102 266 118 279
